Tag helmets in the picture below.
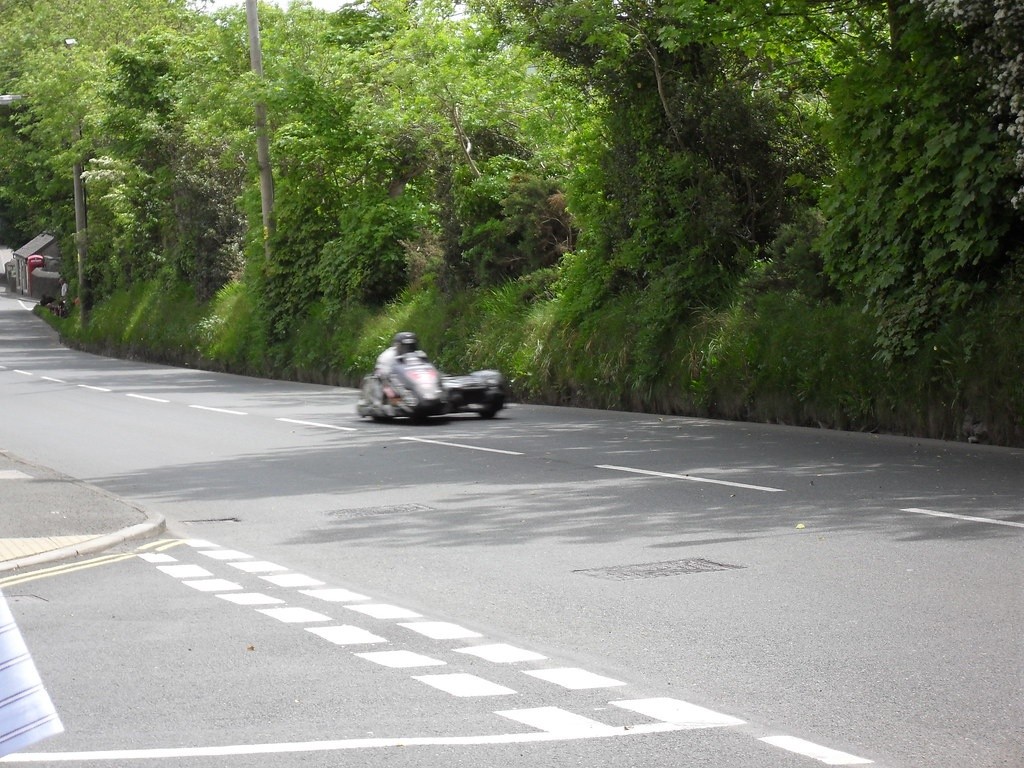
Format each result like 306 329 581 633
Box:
394 332 417 345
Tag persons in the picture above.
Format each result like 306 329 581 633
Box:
59 278 68 299
374 331 428 381
46 302 57 317
